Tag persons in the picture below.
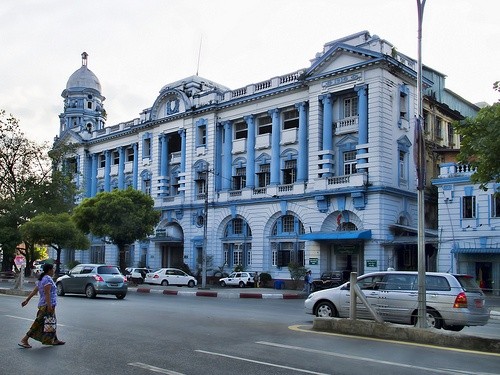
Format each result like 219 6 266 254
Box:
30 268 43 276
18 264 67 348
254 270 313 299
370 277 381 290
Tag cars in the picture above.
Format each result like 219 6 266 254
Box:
145 267 198 288
56 264 128 300
125 267 153 282
219 271 258 287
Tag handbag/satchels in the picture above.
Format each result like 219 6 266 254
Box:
43 311 57 332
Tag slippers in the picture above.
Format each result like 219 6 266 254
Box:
18 342 32 348
53 340 66 345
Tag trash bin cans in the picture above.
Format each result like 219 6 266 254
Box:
274 279 284 290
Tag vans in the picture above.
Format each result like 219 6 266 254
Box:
305 271 491 332
